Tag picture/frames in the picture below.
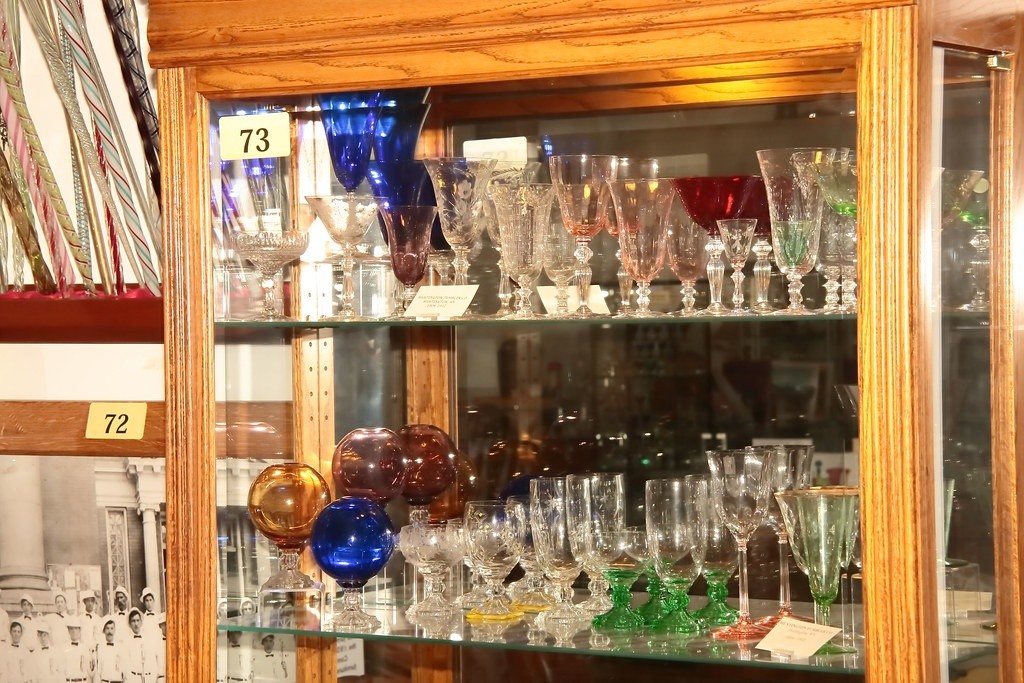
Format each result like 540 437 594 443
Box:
0 398 296 683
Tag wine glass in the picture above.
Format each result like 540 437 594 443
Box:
229 86 858 324
247 424 862 654
943 169 985 313
958 169 998 312
972 466 999 632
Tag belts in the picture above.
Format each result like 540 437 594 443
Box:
101 679 123 683
131 672 142 676
228 676 247 681
158 676 164 678
66 678 87 682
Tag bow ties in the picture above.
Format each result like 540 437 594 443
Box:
11 644 19 647
24 615 31 620
231 644 240 647
71 642 78 646
265 654 274 657
42 646 49 650
119 613 125 615
163 638 166 640
134 635 141 638
86 614 92 619
107 642 114 646
147 613 154 615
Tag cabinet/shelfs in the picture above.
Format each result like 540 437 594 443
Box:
143 0 1024 683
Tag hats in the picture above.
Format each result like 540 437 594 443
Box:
67 619 81 628
19 595 34 610
100 614 115 633
139 587 156 603
127 606 144 628
216 597 227 619
80 590 96 602
156 612 166 626
54 591 67 604
239 597 256 615
36 624 50 637
259 632 275 645
114 585 128 600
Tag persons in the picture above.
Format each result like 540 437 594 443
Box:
215 598 296 683
1 587 167 683
